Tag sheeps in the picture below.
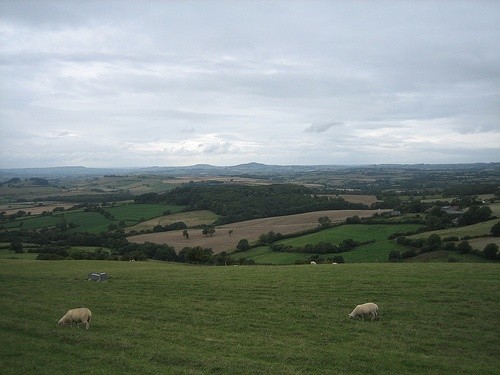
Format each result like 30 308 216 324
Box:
58 308 91 331
348 303 379 322
